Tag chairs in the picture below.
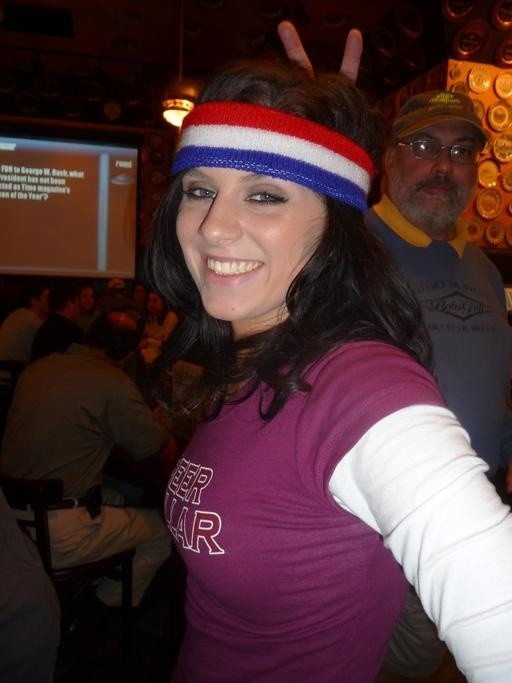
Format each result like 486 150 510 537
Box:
0 474 135 662
0 358 25 408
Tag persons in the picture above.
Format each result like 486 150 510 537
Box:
278 18 512 683
151 59 512 683
1 279 181 683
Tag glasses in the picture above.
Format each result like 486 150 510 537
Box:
396 138 482 165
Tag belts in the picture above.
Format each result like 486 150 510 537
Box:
11 498 83 511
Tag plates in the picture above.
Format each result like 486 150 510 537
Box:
444 62 511 252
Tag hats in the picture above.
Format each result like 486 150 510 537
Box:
393 90 487 153
